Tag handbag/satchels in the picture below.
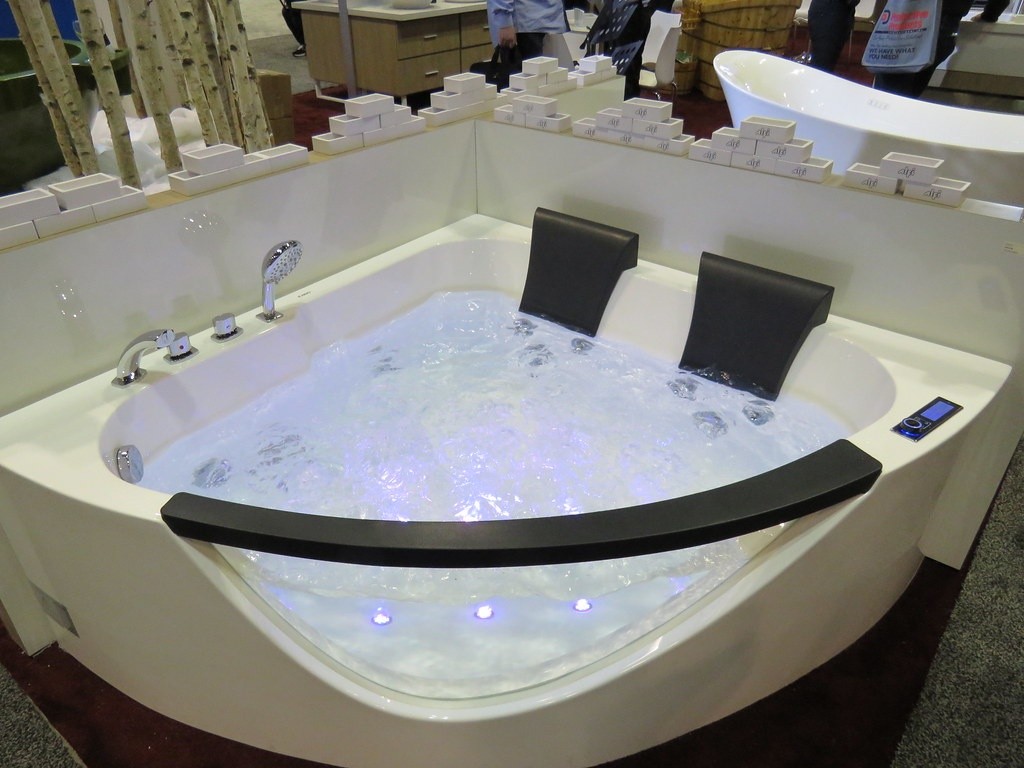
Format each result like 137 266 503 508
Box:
469 44 524 92
860 0 942 74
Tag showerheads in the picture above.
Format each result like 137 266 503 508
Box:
261 239 304 322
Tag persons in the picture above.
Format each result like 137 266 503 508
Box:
279 0 308 57
602 0 651 101
486 0 570 84
866 0 1012 100
808 0 862 74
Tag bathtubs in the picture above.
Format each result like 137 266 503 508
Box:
0 38 133 194
714 48 1024 211
1 211 1013 768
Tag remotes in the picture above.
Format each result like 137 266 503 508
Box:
890 396 964 442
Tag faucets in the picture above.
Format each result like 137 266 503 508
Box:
117 328 176 383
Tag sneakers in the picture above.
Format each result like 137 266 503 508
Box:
293 44 306 57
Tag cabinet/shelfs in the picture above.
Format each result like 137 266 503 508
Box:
300 11 462 96
460 10 502 73
